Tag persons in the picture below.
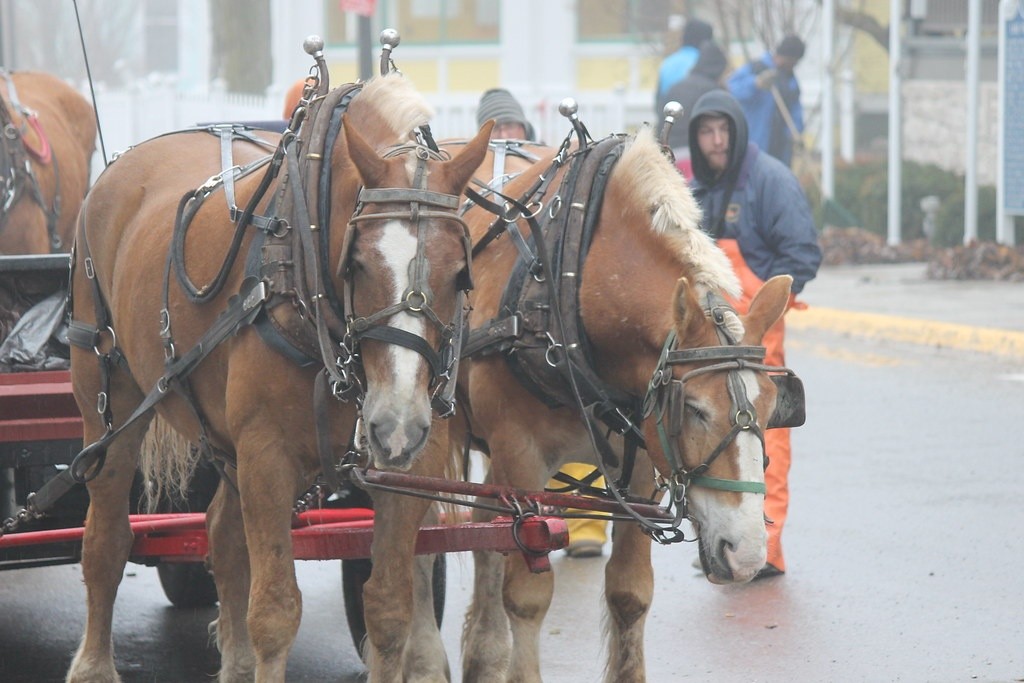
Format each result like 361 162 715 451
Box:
476 88 609 560
655 18 806 188
687 90 822 579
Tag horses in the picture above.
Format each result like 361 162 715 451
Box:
0 71 97 255
398 126 793 683
65 72 496 683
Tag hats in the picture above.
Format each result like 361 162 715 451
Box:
476 88 529 137
778 37 804 58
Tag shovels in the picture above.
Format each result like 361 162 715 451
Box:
748 58 857 230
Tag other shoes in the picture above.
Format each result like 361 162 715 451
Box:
755 557 788 585
565 538 603 557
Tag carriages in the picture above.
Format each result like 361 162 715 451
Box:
0 69 808 683
